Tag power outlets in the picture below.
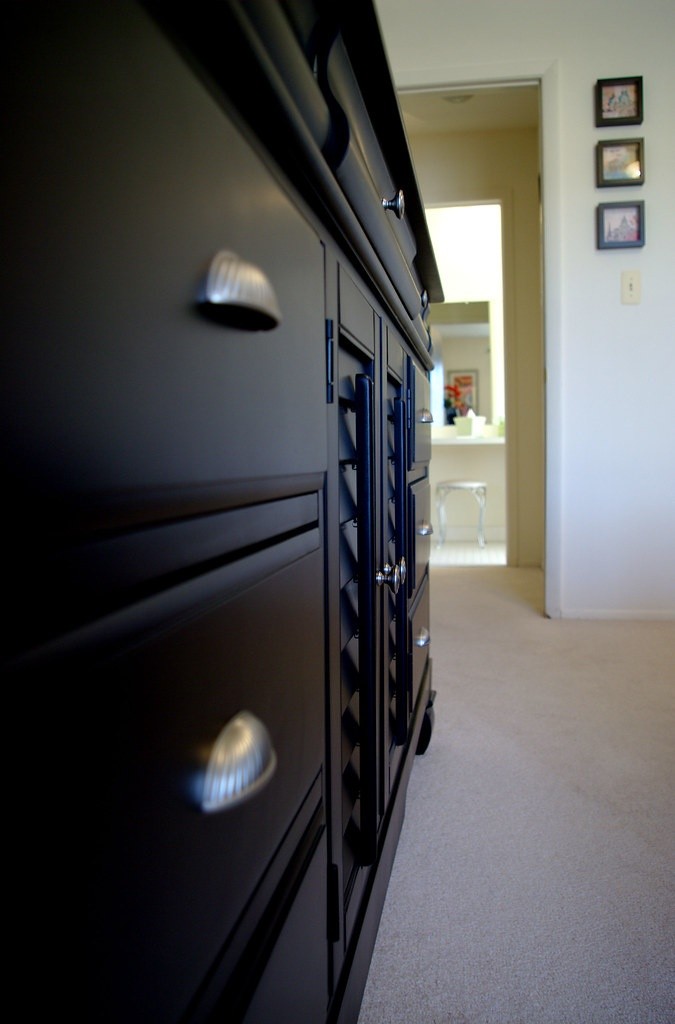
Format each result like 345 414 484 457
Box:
621 269 643 306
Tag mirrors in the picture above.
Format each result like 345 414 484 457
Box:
427 300 495 429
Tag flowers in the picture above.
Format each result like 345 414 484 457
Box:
444 384 465 410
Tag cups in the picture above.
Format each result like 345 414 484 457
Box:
472 416 487 437
453 416 472 437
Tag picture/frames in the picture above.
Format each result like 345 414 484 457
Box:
597 137 646 187
447 368 480 417
594 75 644 127
598 200 647 249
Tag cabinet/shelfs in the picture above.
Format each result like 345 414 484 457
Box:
0 0 445 1024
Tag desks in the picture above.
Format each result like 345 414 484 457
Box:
430 437 505 445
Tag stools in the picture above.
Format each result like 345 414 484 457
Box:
438 482 488 550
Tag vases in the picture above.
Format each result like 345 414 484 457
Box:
446 408 457 425
